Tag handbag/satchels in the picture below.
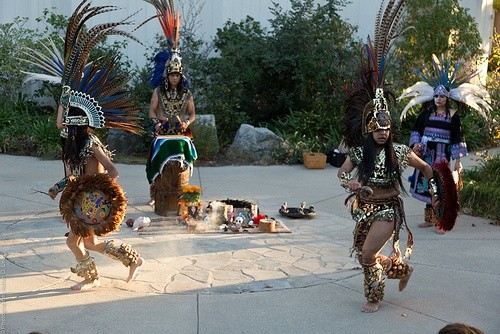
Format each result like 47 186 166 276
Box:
330 151 347 167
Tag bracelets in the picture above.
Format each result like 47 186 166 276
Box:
184 120 190 125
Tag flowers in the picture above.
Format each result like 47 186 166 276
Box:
177 185 203 222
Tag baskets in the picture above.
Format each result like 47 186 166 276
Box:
304 153 327 169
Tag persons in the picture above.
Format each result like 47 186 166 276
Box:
337 87 441 313
145 48 196 206
408 84 466 235
47 87 143 292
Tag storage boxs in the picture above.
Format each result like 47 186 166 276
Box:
303 153 327 168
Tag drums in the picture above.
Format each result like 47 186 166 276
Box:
153 132 192 191
